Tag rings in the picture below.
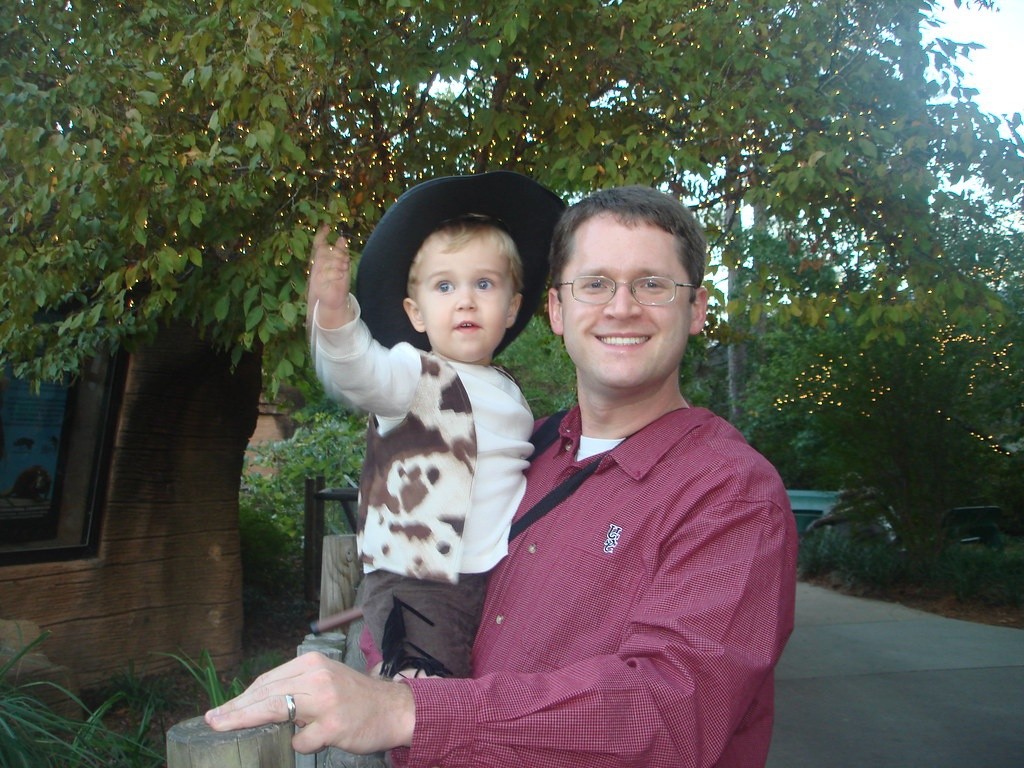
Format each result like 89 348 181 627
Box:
286 694 297 722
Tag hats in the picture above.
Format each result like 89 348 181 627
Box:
353 170 568 363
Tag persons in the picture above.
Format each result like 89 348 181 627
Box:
206 183 796 768
306 217 535 682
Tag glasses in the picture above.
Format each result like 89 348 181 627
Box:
557 275 697 307
